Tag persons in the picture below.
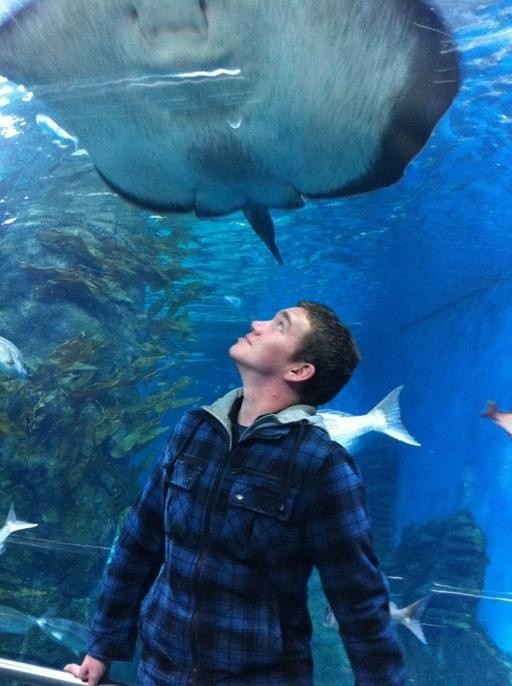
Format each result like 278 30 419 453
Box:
61 300 408 685
